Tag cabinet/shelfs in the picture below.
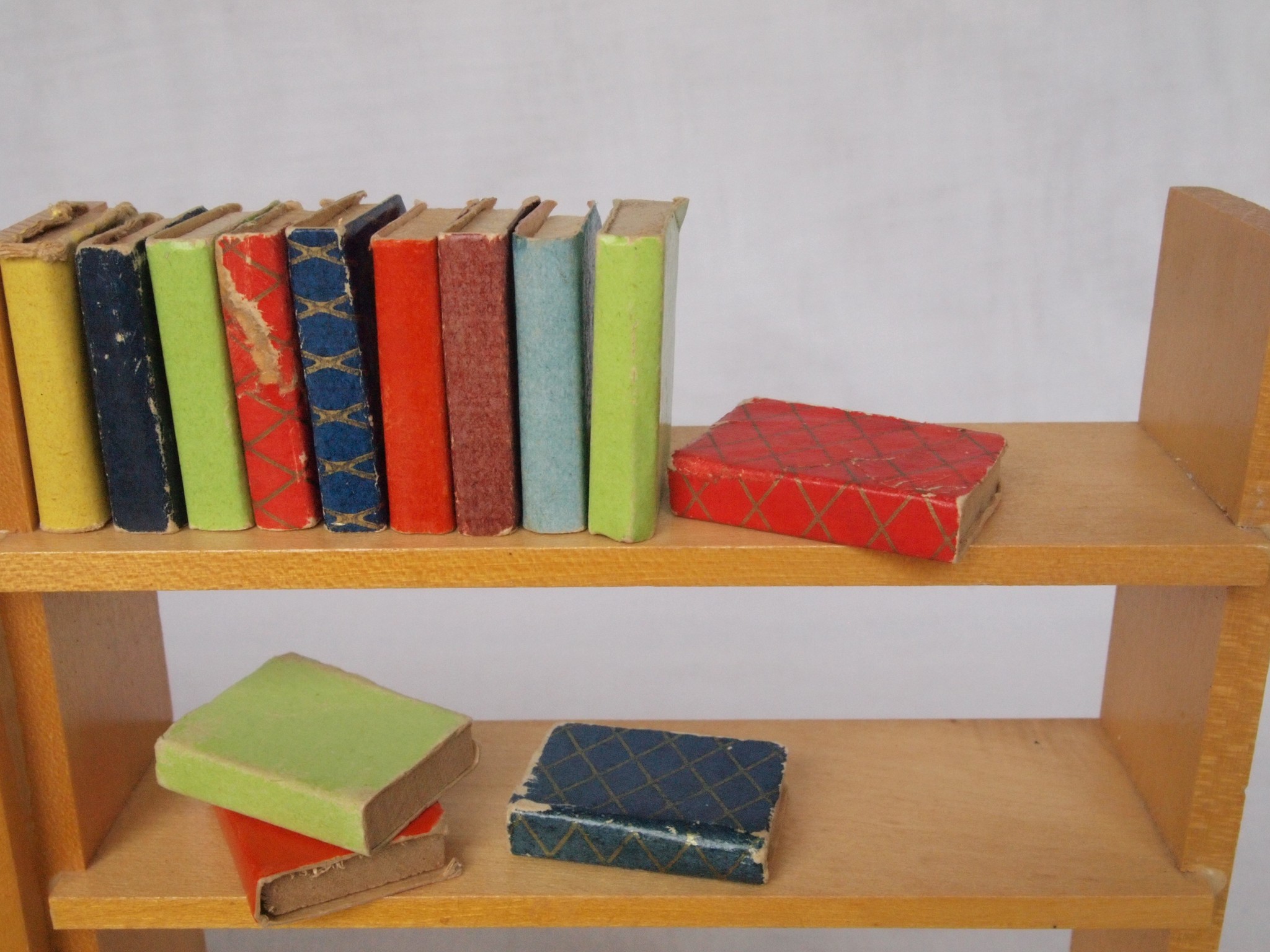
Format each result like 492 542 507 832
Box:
0 185 1270 952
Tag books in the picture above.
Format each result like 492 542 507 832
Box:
211 804 466 927
153 652 479 856
665 398 1007 564
506 722 788 884
0 200 689 541
